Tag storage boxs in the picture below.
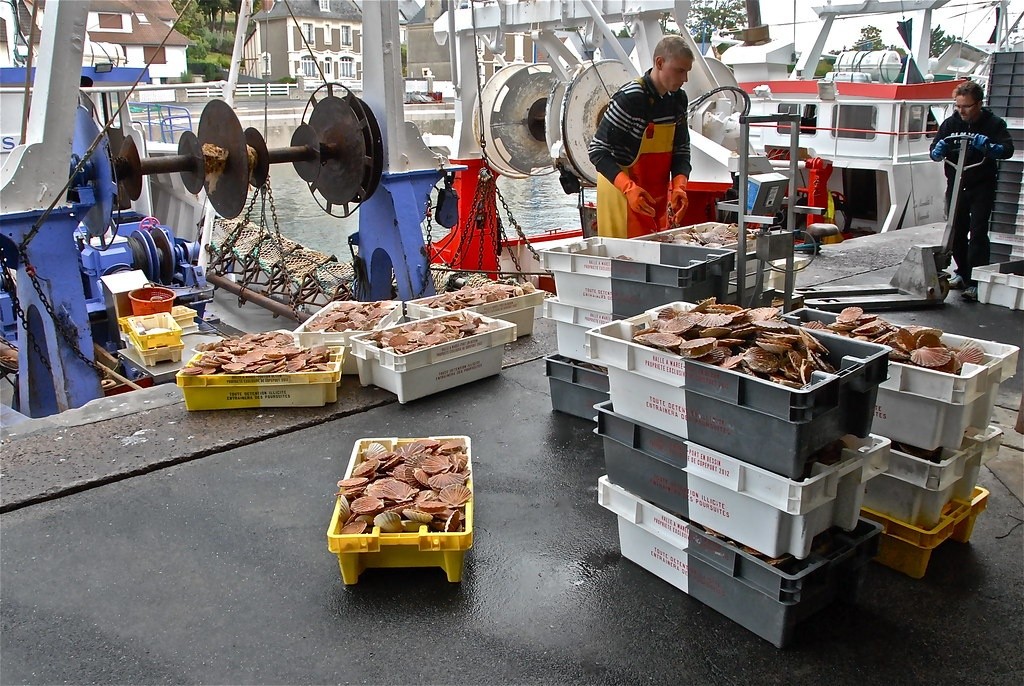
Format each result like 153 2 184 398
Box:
126 312 182 350
129 339 185 367
597 475 886 649
970 48 1024 310
541 296 629 367
782 307 1021 450
293 300 404 374
859 485 990 579
175 345 346 412
542 352 611 421
592 400 892 559
327 436 473 584
583 300 893 479
539 236 739 316
862 425 1004 528
120 322 200 348
630 222 806 308
349 310 517 404
118 305 198 334
403 283 545 338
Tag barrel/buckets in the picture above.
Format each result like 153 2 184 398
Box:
128 282 176 317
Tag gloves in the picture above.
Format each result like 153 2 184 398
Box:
972 133 1003 159
614 172 656 217
671 174 688 223
932 140 948 161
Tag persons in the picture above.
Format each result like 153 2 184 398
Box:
588 34 696 240
929 81 1015 301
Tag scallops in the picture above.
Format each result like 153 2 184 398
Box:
333 439 472 533
181 280 537 375
633 296 984 387
648 214 760 247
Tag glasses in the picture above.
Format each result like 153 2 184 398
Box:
955 102 976 108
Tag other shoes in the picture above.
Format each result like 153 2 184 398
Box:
961 286 977 300
948 270 966 289
